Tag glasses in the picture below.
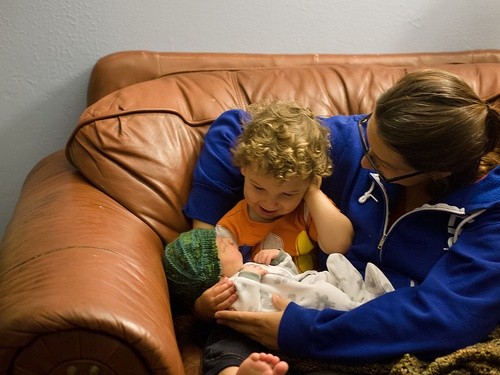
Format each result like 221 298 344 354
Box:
357 109 434 186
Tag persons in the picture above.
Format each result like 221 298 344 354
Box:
192 100 353 375
186 68 500 364
165 227 393 322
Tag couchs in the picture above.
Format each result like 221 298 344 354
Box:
0 49 500 375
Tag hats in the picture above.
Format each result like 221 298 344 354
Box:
161 228 222 297
